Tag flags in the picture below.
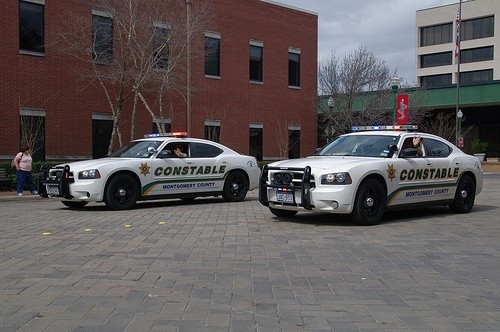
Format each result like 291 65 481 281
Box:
454 5 460 58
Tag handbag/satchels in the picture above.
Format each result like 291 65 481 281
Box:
10 160 19 172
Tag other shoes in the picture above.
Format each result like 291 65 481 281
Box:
31 190 38 195
16 191 22 196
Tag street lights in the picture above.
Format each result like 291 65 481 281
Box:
327 96 335 142
390 73 400 126
457 110 464 151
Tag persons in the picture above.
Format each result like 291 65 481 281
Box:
14 145 38 195
166 144 187 158
400 136 423 156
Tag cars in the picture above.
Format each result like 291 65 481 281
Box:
37 132 262 210
259 124 484 227
